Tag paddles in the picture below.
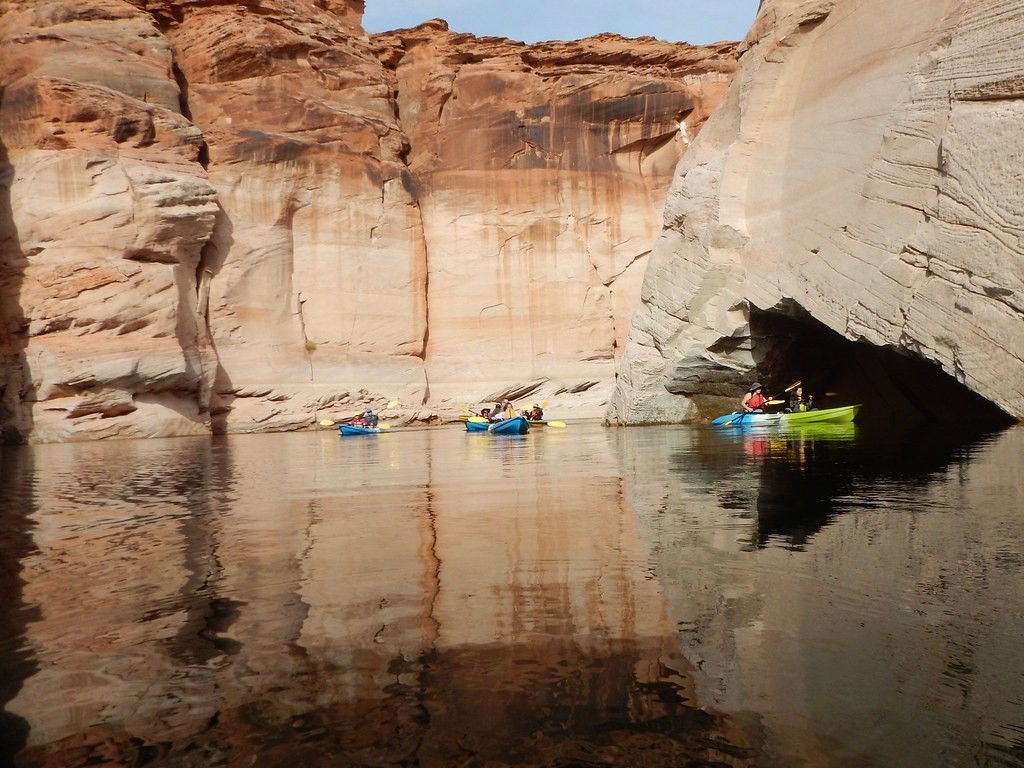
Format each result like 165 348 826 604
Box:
468 417 566 428
514 403 534 411
766 392 840 405
458 415 472 421
724 380 802 425
462 405 481 417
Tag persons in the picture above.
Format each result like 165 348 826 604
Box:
741 382 773 412
787 386 812 412
529 403 543 420
349 409 379 427
490 398 523 424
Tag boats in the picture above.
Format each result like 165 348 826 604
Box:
338 424 385 438
714 420 860 444
462 419 491 432
490 415 531 433
711 403 864 425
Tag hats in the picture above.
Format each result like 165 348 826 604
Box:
750 383 762 391
532 403 540 409
367 409 372 413
481 408 490 414
